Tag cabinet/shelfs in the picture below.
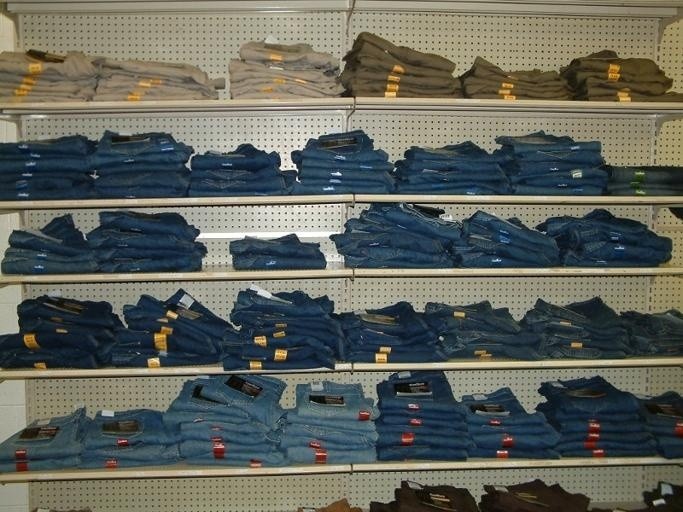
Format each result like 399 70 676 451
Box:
2 0 683 512
